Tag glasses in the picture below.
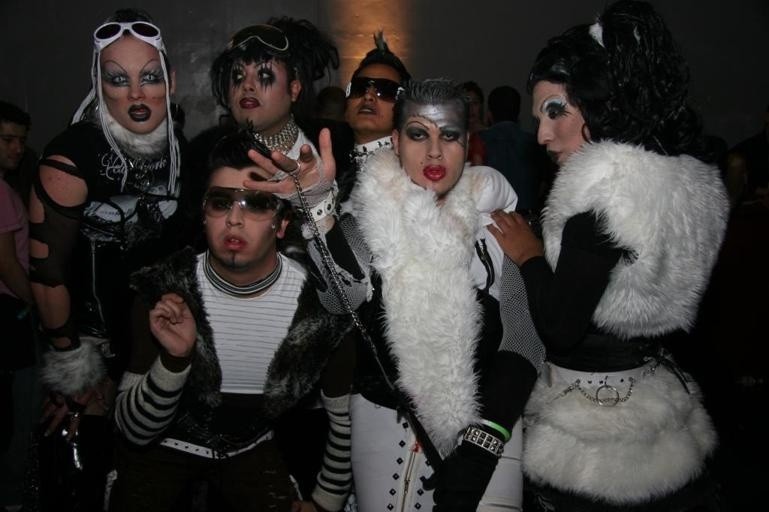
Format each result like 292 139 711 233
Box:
205 184 283 220
232 24 290 50
91 20 167 59
344 78 403 102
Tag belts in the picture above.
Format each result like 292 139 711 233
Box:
159 431 275 461
540 352 659 389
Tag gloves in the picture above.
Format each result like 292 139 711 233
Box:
423 448 498 512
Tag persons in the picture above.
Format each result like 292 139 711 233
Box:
1 0 769 511
